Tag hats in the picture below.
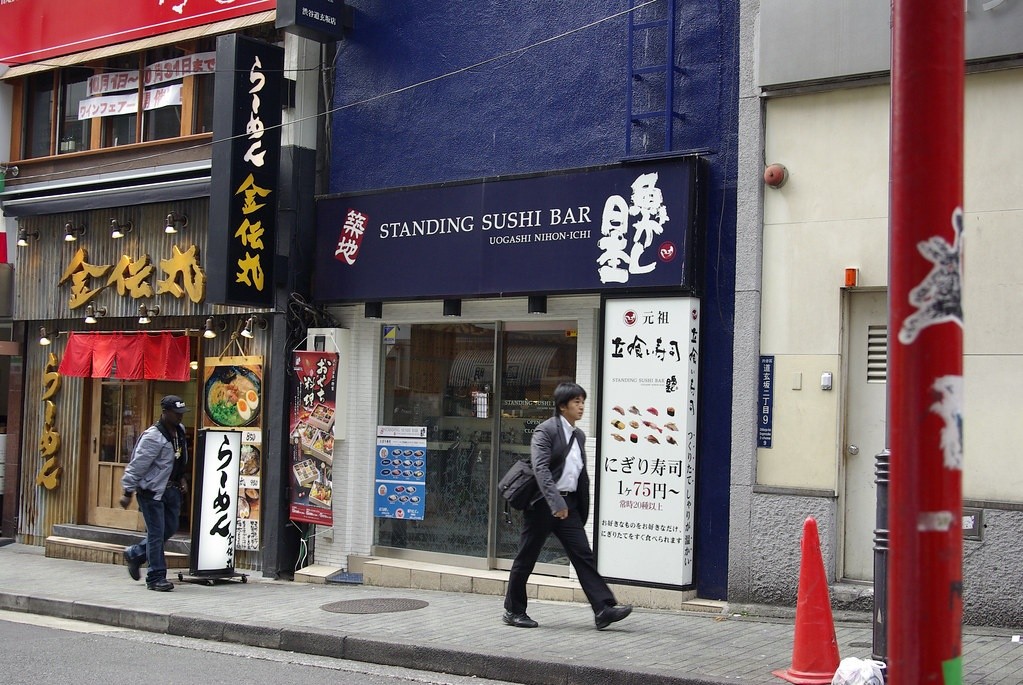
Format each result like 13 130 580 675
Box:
161 395 191 414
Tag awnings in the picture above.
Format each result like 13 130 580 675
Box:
449 344 560 388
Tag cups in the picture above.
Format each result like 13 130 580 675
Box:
845 267 856 286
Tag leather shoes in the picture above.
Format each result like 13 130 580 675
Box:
147 578 174 591
124 547 140 580
502 609 538 628
595 604 631 630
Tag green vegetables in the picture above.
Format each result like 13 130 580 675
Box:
241 444 254 452
210 400 239 425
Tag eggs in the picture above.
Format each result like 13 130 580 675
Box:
240 508 249 519
236 391 258 419
240 461 244 471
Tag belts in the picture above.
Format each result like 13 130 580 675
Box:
559 491 578 497
166 481 182 491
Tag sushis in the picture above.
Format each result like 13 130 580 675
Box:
612 405 679 447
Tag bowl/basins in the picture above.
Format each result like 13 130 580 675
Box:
203 366 261 427
236 446 261 519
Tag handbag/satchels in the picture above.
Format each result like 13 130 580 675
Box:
498 461 538 511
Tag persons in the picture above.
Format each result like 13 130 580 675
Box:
119 395 187 590
499 381 633 630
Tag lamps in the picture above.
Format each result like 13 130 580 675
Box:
39 325 61 345
16 229 41 247
84 301 108 325
239 316 268 338
137 303 161 325
110 219 135 239
203 315 227 338
165 214 189 235
63 223 86 242
